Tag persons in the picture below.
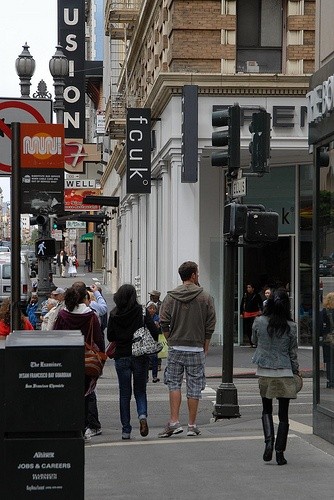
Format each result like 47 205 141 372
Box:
263 288 271 307
250 288 299 464
319 293 334 387
240 284 263 347
159 261 216 437
0 249 169 443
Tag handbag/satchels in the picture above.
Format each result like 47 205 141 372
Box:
157 333 168 358
106 341 117 359
132 304 164 356
85 316 107 377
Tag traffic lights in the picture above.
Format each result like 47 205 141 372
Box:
223 202 247 235
53 217 58 229
30 214 49 225
211 105 241 169
34 238 56 258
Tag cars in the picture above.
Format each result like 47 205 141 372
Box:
0 239 39 317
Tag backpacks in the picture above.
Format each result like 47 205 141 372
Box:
317 308 330 337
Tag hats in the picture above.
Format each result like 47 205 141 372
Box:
53 288 65 294
148 290 161 296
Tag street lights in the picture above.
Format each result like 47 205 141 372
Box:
13 40 69 331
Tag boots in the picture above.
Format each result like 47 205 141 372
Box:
275 422 290 465
262 414 275 462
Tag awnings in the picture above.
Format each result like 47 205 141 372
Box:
80 232 94 242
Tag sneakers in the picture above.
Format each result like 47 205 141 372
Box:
187 426 200 435
157 420 183 438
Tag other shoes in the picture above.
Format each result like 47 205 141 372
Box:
122 433 130 440
157 365 161 371
140 414 148 437
91 425 103 436
327 381 334 388
153 378 160 383
83 428 91 442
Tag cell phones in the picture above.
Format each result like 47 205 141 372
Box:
86 286 93 292
